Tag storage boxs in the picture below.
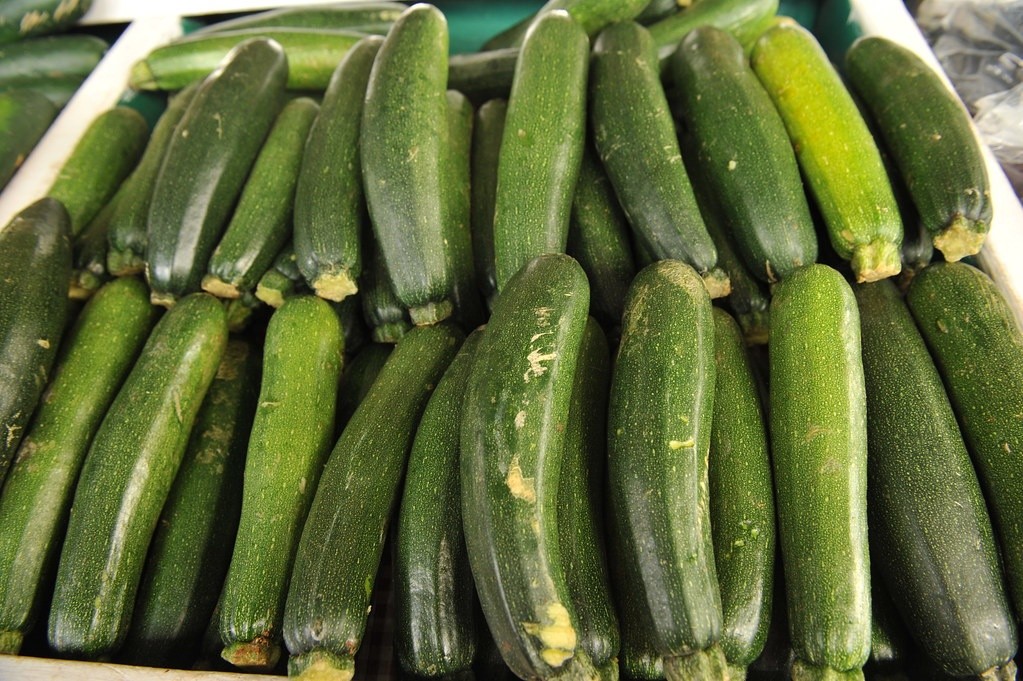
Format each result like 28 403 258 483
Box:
0 0 1023 354
